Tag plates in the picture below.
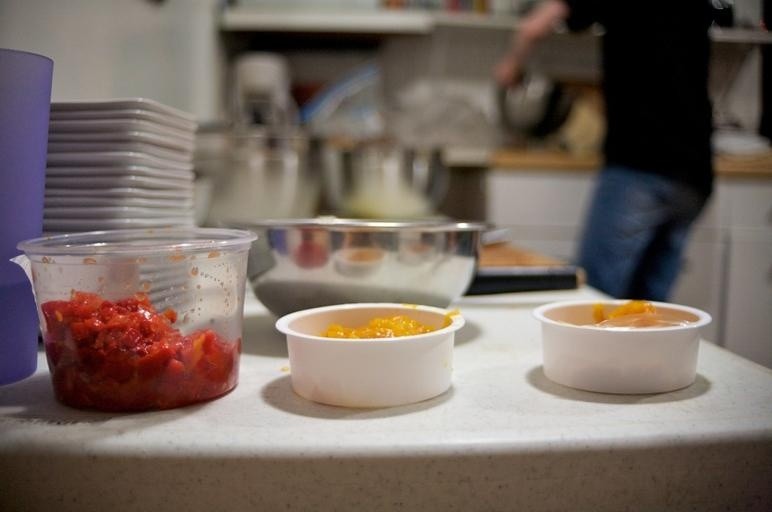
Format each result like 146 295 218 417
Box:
47 95 200 314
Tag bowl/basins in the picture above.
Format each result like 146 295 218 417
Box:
202 216 487 317
316 66 577 212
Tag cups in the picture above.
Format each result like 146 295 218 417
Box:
0 48 57 390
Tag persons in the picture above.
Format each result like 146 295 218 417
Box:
489 0 737 303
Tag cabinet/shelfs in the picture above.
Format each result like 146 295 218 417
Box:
452 165 772 368
